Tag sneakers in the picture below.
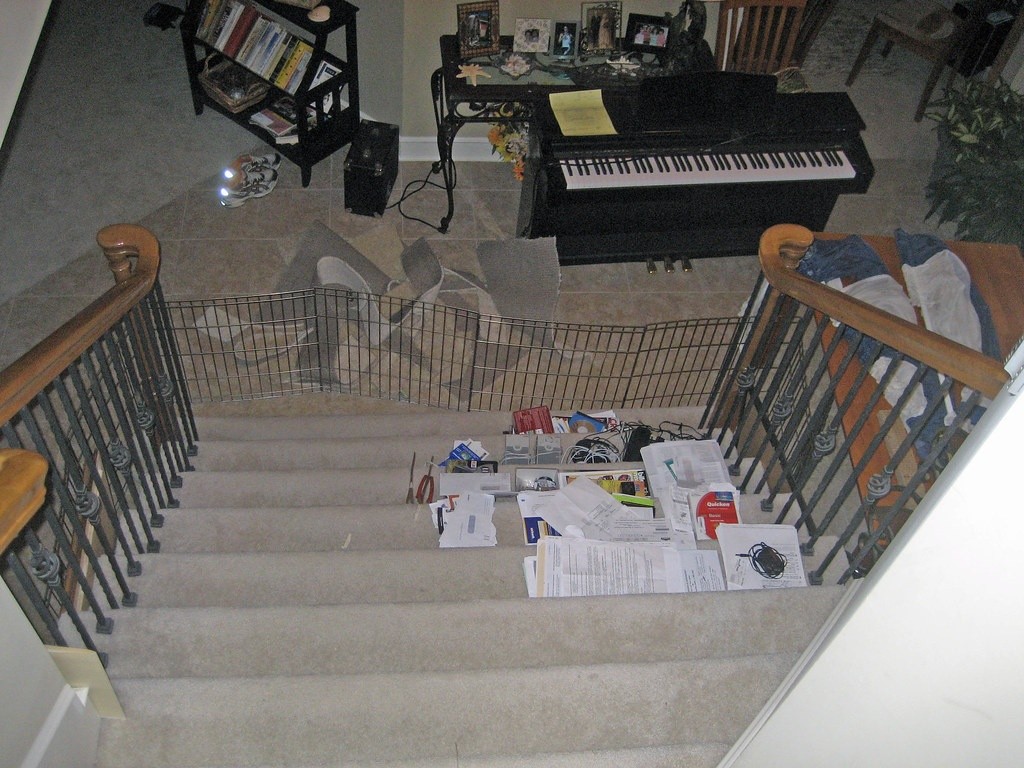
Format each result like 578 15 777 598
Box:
215 166 279 209
227 153 282 171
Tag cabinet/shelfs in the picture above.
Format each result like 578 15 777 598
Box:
178 0 361 190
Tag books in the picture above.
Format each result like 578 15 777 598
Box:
196 0 343 144
431 406 742 540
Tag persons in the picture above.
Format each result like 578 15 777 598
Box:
591 10 612 48
558 25 573 55
525 30 538 43
636 24 665 46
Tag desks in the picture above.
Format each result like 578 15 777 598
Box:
762 231 1024 571
431 36 720 230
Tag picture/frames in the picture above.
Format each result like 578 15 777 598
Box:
457 0 500 59
581 1 622 59
549 19 579 59
624 13 673 55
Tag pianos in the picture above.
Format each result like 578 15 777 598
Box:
511 69 880 278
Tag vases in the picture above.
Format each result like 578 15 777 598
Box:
926 147 1002 223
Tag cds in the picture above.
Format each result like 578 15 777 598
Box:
570 420 594 434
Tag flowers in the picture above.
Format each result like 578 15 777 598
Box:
921 72 1024 258
486 101 531 180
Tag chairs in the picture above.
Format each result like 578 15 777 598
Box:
715 0 807 74
846 0 995 123
